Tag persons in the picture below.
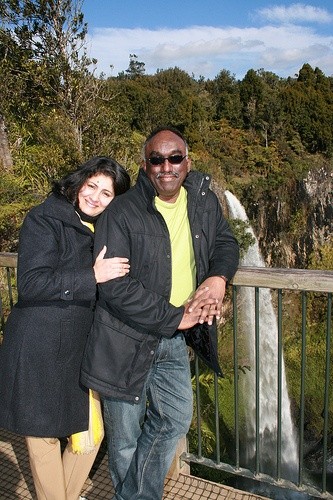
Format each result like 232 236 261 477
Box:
0 156 132 500
80 125 240 500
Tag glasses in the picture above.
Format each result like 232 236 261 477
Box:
147 155 187 165
